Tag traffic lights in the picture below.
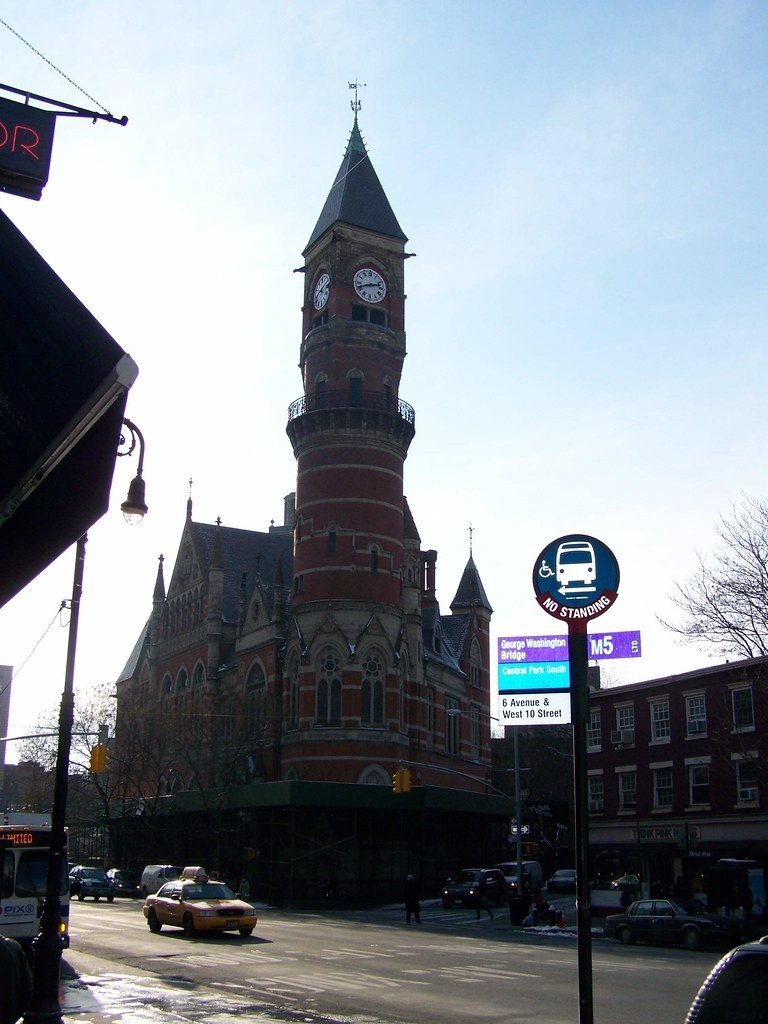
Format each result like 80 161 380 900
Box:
88 745 110 773
393 771 402 794
403 771 412 792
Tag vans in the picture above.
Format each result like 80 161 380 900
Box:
140 865 184 898
547 869 577 894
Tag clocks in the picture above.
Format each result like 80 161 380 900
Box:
313 273 330 310
353 267 386 304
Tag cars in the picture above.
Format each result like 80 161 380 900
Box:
605 898 741 950
683 935 768 1024
68 862 141 902
610 875 640 891
143 866 257 937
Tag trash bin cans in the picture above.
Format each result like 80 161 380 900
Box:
510 895 529 925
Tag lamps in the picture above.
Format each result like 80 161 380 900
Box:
120 418 148 527
165 768 180 795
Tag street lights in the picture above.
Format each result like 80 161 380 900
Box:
446 709 530 928
23 418 148 1024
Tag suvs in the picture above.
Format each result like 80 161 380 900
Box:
494 862 546 895
442 868 509 908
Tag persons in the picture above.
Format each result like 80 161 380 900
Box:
405 874 424 925
475 877 494 919
238 878 250 902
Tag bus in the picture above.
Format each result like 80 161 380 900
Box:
0 825 81 949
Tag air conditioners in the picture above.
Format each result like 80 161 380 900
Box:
739 787 757 802
589 799 603 811
610 729 635 744
686 719 707 733
